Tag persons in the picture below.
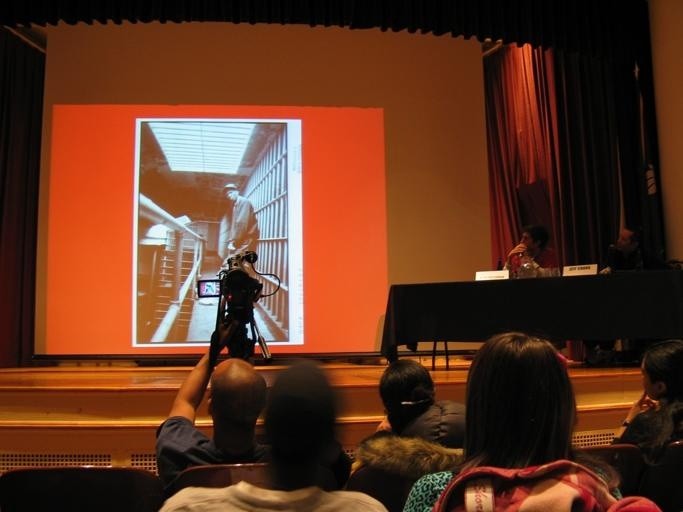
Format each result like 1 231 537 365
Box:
152 313 272 481
607 336 683 447
153 358 394 512
582 224 672 363
504 225 573 363
398 327 622 512
379 359 470 450
215 184 262 272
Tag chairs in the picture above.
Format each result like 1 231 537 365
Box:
343 463 419 511
0 460 273 512
575 441 682 512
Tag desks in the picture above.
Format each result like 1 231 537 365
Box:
390 266 682 370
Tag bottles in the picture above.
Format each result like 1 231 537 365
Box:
517 252 536 279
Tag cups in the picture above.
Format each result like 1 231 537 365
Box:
540 268 560 276
